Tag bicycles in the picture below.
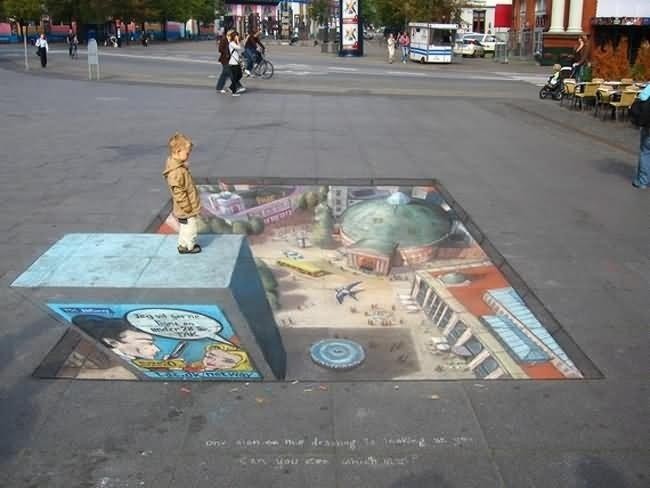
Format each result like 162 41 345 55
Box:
252 58 268 76
238 48 275 80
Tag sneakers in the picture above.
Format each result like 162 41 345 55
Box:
215 89 225 94
235 87 246 93
244 68 251 75
177 245 201 253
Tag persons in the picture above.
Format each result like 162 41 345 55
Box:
398 31 410 63
142 33 149 48
33 32 50 66
162 130 201 254
569 35 589 74
549 63 562 86
200 341 256 372
104 31 111 49
65 28 79 56
109 32 119 48
71 313 162 363
386 32 397 64
629 82 650 189
213 27 266 97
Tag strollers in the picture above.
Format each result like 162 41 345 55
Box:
537 57 579 102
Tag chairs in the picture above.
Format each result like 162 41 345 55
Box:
562 77 646 127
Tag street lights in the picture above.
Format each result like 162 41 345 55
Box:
8 15 31 71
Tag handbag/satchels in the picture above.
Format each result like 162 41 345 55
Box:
37 47 41 56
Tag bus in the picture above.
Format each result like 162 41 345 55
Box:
276 256 322 280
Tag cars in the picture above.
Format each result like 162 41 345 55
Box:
363 30 374 40
462 33 504 55
283 249 305 259
453 39 485 58
